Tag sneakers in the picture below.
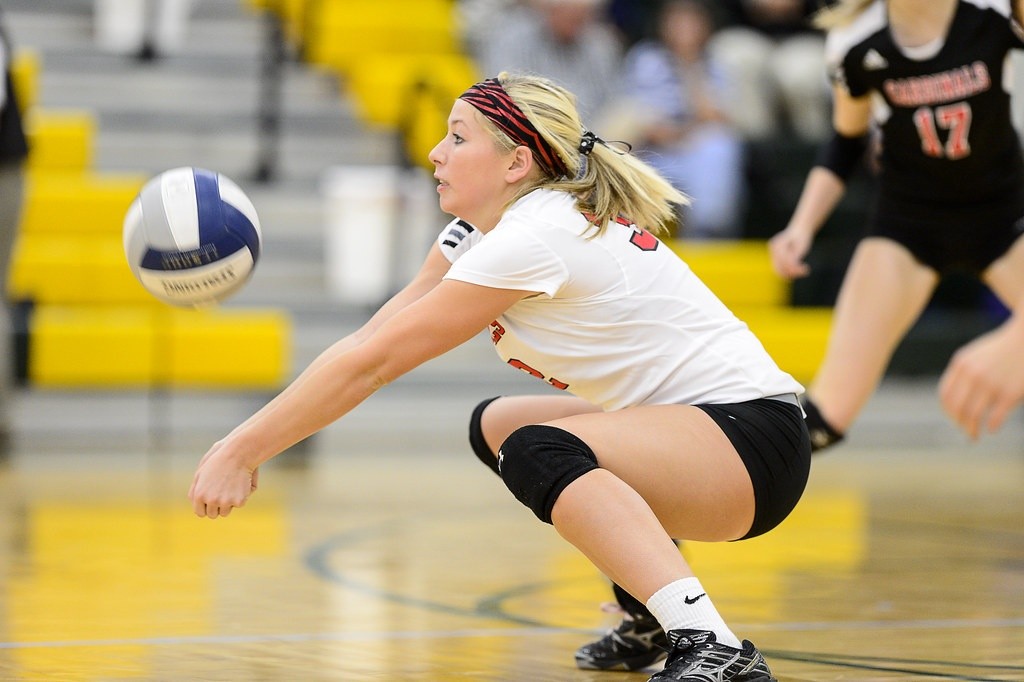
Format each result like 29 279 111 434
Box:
645 627 778 682
575 582 670 671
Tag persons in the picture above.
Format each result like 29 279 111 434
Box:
194 72 812 682
768 2 1022 453
0 32 35 452
468 0 833 237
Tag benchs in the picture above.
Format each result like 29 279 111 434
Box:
1 1 993 398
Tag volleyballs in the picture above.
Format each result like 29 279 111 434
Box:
121 167 261 306
